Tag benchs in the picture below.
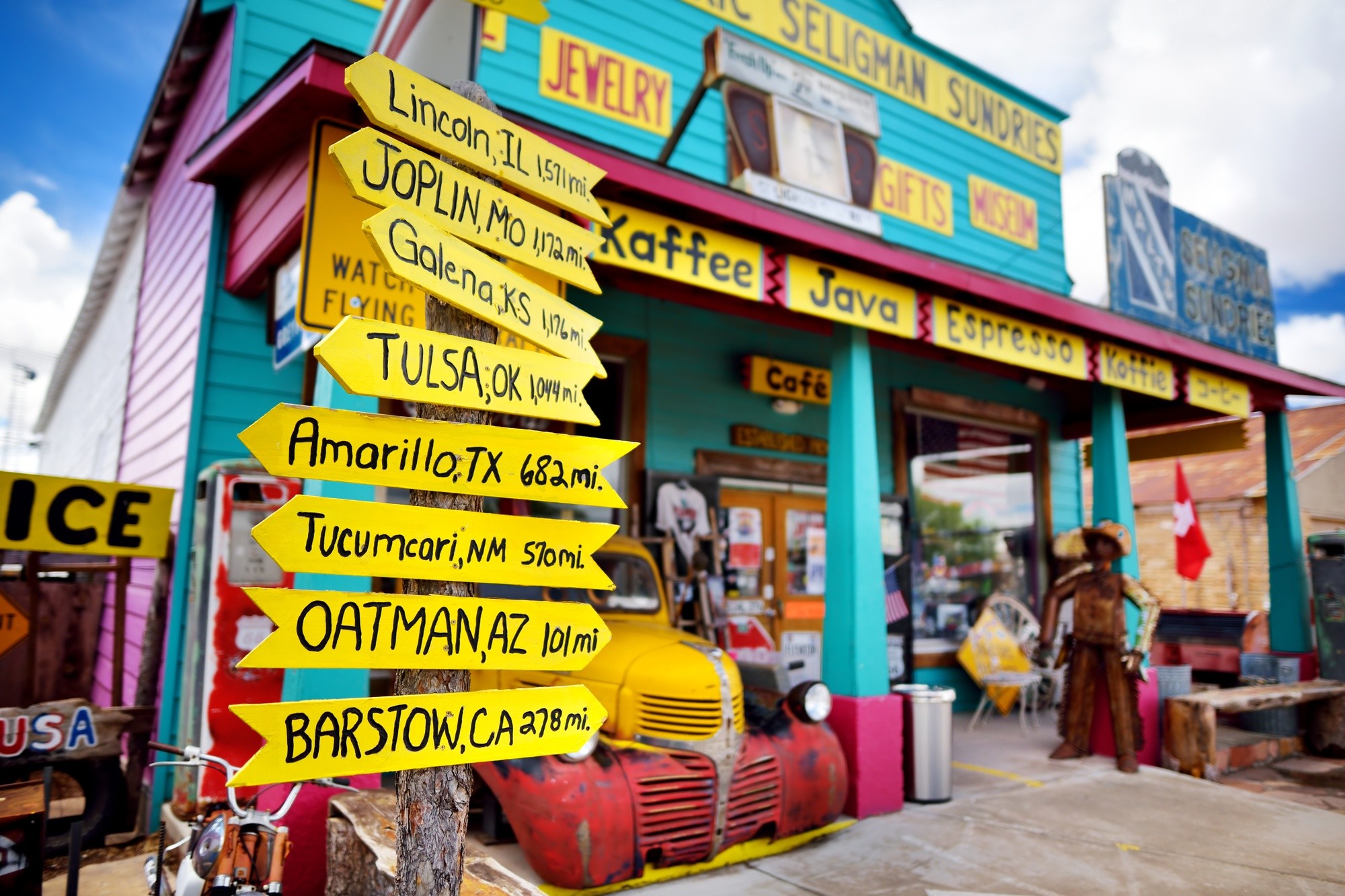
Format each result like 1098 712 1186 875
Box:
1162 680 1345 783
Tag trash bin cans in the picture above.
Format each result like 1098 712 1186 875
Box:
894 684 956 804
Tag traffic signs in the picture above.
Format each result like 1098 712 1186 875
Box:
238 402 642 510
345 50 616 230
225 682 610 789
238 586 613 672
251 492 621 591
361 198 608 380
330 126 608 295
316 315 600 429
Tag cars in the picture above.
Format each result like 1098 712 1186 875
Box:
371 524 860 895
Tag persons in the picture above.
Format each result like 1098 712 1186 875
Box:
1040 524 1161 773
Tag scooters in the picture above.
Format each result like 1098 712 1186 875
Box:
143 742 351 896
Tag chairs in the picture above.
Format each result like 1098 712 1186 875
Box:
969 595 1041 731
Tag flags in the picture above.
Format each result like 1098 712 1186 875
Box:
1173 461 1213 581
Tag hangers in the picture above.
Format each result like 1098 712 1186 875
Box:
678 479 690 489
